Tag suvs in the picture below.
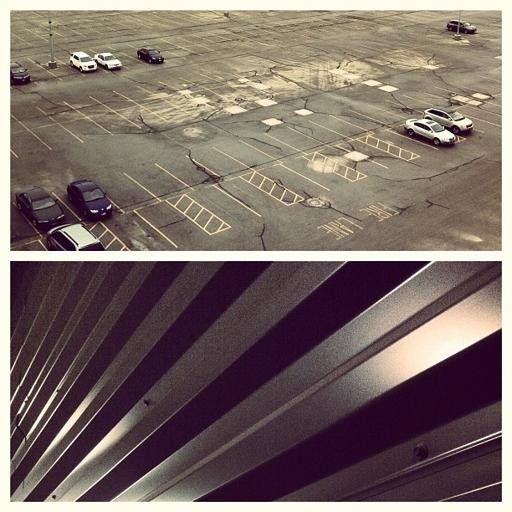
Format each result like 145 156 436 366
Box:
70 51 97 73
46 221 104 251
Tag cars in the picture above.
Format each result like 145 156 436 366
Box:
10 62 30 85
447 19 477 34
67 179 112 220
424 105 474 134
403 118 456 146
137 48 164 64
15 185 65 228
94 53 122 70
